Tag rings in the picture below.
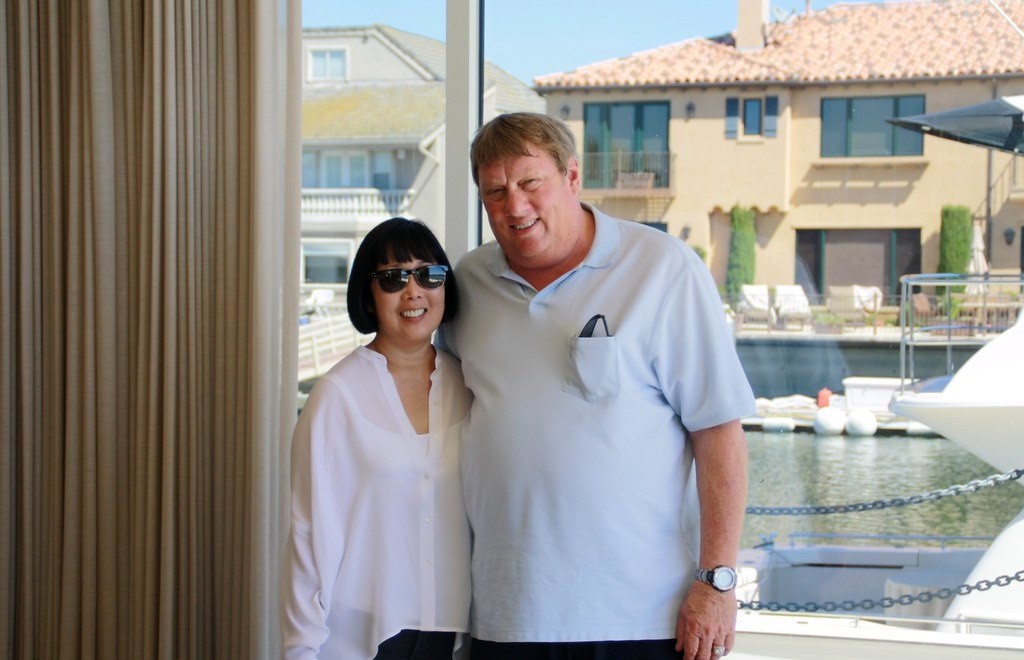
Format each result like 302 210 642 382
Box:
713 646 725 656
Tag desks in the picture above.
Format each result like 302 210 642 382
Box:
959 301 1023 334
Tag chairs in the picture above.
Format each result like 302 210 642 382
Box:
737 284 1023 333
305 289 335 319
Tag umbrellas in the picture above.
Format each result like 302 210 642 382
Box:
884 94 1023 159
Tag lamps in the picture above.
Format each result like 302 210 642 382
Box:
685 101 695 118
560 103 569 119
1004 227 1015 245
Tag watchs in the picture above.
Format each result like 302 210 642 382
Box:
694 564 738 593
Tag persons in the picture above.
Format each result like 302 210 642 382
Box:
281 217 474 660
433 112 758 660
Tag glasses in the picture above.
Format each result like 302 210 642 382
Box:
370 265 448 294
571 314 612 400
